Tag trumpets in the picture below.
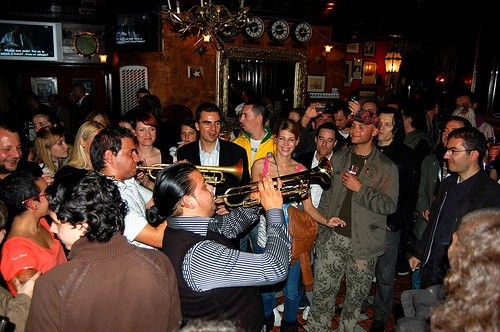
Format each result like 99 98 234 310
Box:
213 156 334 212
135 158 244 186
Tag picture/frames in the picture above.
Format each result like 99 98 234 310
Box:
357 88 377 101
307 73 326 93
353 58 363 80
342 60 354 86
362 61 377 84
72 77 98 100
363 39 376 57
31 77 58 107
344 42 360 54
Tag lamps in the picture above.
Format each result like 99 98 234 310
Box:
166 0 251 43
314 45 333 62
384 39 404 92
98 48 109 64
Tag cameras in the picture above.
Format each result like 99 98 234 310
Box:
316 104 326 112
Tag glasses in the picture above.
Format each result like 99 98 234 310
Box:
21 190 49 205
444 146 476 156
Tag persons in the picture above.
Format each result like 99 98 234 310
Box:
230 100 278 175
24 170 182 332
451 89 498 181
411 115 472 289
368 107 409 331
178 102 250 217
0 169 68 295
384 90 439 276
410 127 500 289
180 119 197 144
89 126 167 249
145 163 287 332
0 83 110 183
0 202 42 332
288 100 383 169
119 87 194 191
249 119 346 332
303 109 400 332
396 208 500 332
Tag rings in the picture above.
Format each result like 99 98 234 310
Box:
278 188 282 190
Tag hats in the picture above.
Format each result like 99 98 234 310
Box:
353 109 380 129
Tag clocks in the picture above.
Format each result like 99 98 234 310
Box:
267 18 291 41
243 17 265 39
292 21 313 45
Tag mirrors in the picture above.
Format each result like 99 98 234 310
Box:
73 31 99 58
214 47 308 123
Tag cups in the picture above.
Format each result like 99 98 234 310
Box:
490 144 498 160
15 268 38 284
346 165 359 175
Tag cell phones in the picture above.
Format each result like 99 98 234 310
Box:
350 95 356 102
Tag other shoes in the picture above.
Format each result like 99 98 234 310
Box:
398 267 409 275
273 308 282 327
369 320 385 332
367 295 375 304
263 313 275 332
281 318 299 332
276 303 285 312
334 306 365 315
302 305 310 320
